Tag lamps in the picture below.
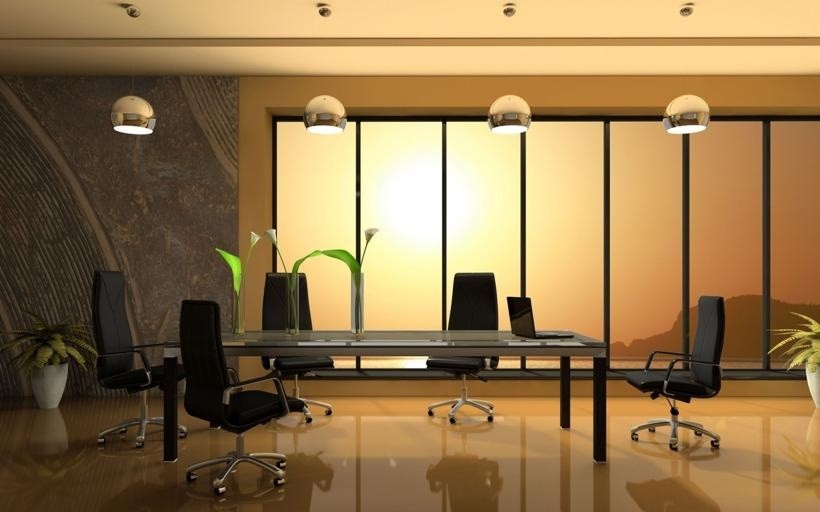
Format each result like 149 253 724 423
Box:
663 95 710 135
488 95 530 134
303 95 346 134
111 95 156 135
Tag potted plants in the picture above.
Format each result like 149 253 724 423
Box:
266 227 322 340
214 230 262 337
0 311 99 408
0 408 98 495
322 227 378 336
764 408 820 498
767 312 820 410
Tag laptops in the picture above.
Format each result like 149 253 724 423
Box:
507 296 574 339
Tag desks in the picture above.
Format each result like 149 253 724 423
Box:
164 329 606 466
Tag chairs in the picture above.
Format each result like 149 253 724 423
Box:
625 478 721 512
181 299 308 496
91 269 188 452
426 457 501 512
259 450 334 512
259 273 333 423
627 297 725 451
425 272 499 425
